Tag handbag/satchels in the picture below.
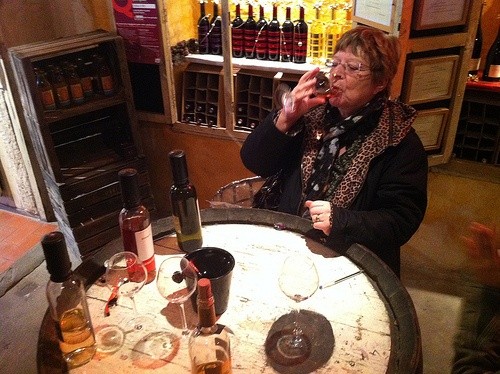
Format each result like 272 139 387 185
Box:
253 179 282 211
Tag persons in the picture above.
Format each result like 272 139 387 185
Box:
239 26 429 280
449 222 500 374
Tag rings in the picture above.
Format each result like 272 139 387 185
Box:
315 214 320 222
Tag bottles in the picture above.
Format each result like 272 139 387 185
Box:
33 46 118 111
467 3 483 76
197 1 209 54
244 4 256 58
209 2 221 55
183 103 218 126
235 106 258 128
167 149 202 253
116 168 156 285
482 27 500 82
189 279 232 374
256 2 353 65
40 230 97 367
231 3 244 57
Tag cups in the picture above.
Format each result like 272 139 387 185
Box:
180 247 236 316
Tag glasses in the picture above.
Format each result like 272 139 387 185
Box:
326 54 371 74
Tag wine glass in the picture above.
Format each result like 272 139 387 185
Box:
156 256 197 346
275 67 346 114
278 254 319 348
105 251 154 341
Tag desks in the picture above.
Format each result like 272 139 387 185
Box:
37 207 424 374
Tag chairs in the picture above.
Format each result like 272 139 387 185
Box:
208 176 264 205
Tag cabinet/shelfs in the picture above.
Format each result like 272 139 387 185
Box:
451 79 500 165
156 0 357 205
